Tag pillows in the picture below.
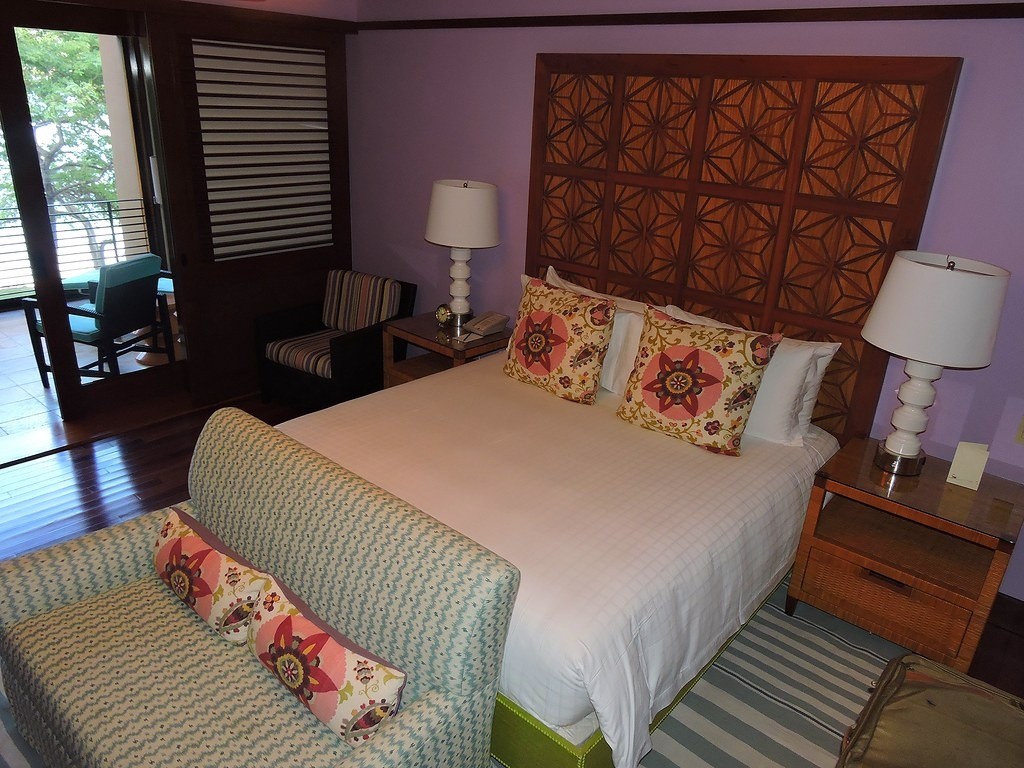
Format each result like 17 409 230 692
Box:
154 508 408 750
664 305 842 447
615 303 785 457
503 274 616 405
547 266 644 395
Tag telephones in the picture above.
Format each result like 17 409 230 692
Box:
463 310 510 336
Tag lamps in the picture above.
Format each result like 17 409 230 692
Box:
424 179 503 327
860 250 1012 475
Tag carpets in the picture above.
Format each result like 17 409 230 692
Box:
0 563 960 768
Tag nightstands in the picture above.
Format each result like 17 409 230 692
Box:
383 309 514 388
786 436 1024 674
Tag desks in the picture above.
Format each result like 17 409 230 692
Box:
135 294 180 365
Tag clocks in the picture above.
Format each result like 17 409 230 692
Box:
435 304 453 323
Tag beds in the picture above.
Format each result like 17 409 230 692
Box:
271 350 843 768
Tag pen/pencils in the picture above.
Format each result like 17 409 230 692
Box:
463 332 472 341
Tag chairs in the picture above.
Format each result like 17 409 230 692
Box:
22 253 176 389
158 270 175 300
255 269 417 408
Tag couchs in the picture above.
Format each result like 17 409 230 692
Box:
0 407 521 768
